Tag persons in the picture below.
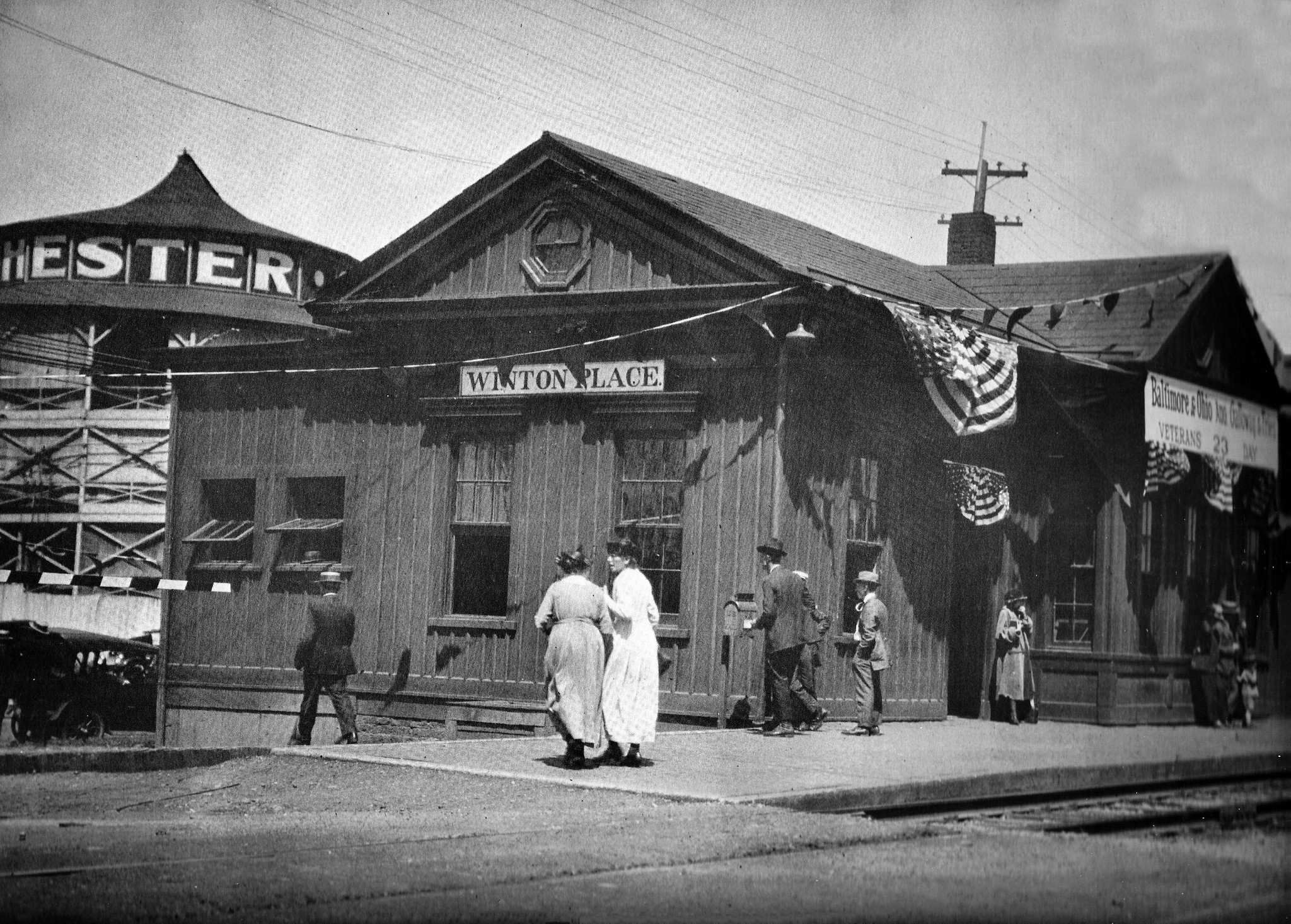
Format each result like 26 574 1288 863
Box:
597 539 661 767
295 571 358 745
1192 600 1259 728
841 571 891 736
995 590 1038 725
750 537 830 737
533 552 614 767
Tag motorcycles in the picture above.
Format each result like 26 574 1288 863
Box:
0 619 160 744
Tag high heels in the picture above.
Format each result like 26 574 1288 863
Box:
592 748 623 763
624 754 641 767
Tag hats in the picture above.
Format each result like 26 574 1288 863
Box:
313 570 343 584
1004 590 1027 603
561 549 593 569
756 537 786 556
853 571 882 588
1221 601 1240 615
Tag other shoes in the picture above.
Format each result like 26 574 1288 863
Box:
564 750 585 769
799 722 809 730
841 726 869 736
810 709 829 731
1223 719 1231 727
869 725 880 734
764 721 793 737
1009 715 1020 725
1213 719 1224 729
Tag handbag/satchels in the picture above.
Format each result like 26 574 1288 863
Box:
1189 651 1215 673
659 650 673 675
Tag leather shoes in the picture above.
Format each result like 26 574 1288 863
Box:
335 733 358 745
287 739 310 745
